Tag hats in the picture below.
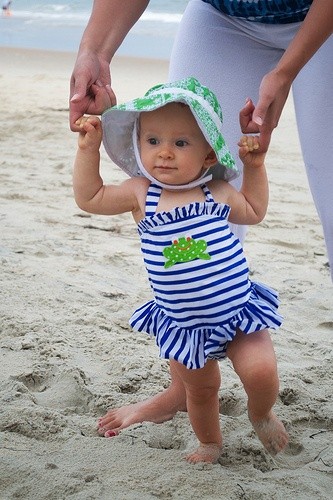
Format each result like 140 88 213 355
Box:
95 74 241 186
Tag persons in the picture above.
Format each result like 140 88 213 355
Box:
67 0 333 438
72 77 290 465
